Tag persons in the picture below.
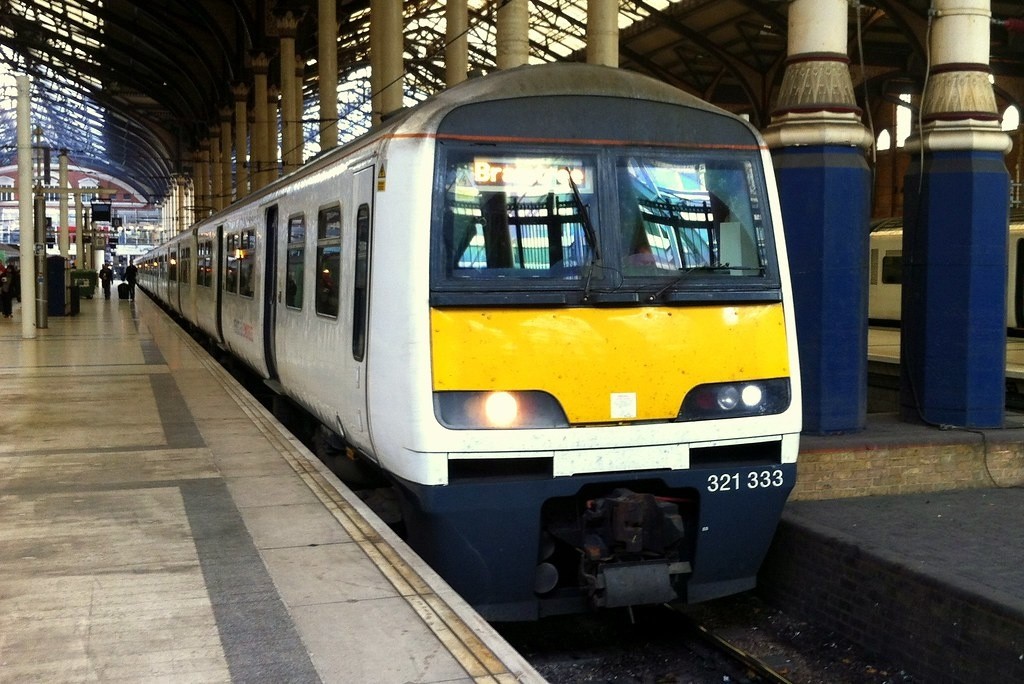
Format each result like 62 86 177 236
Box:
98 262 138 302
0 258 21 317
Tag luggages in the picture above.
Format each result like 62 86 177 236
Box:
118 276 130 300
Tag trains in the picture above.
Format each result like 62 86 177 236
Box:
130 64 806 627
868 220 1024 321
